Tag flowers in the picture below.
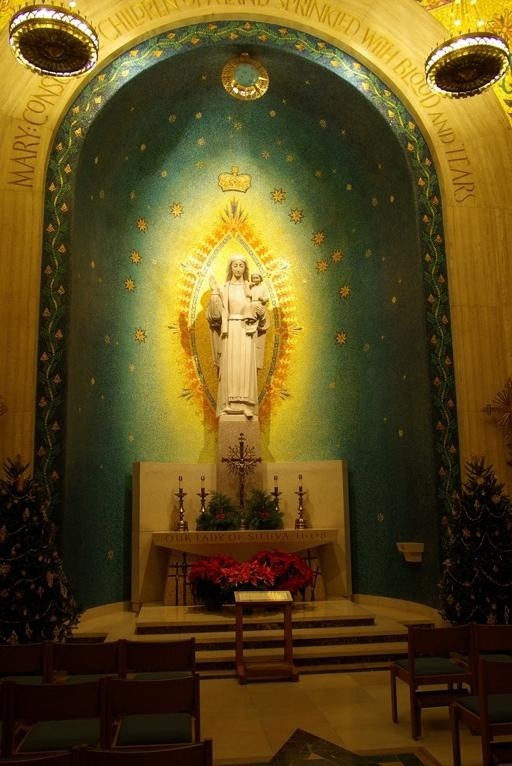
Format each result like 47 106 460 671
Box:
188 553 239 586
240 546 311 589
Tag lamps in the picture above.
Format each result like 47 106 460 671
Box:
425 1 511 101
8 0 101 78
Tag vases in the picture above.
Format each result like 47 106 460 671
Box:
203 588 225 611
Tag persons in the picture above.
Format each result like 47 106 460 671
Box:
239 270 270 331
204 250 266 422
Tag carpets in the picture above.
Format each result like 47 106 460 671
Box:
214 728 444 766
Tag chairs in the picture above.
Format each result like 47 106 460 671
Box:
1 633 215 766
388 624 511 766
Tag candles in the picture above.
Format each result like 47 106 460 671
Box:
298 473 303 490
273 475 278 490
178 475 182 488
200 475 204 490
